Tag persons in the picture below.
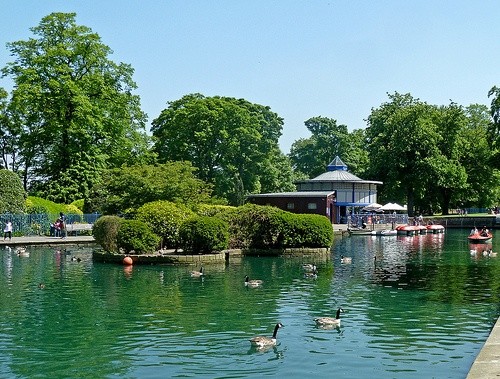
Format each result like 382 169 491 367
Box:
4 220 12 241
54 212 67 239
469 225 488 237
367 212 424 226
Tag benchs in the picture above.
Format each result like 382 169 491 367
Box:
62 224 94 237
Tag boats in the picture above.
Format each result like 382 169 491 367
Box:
380 222 397 236
468 231 493 244
347 216 377 235
396 225 426 235
423 220 446 233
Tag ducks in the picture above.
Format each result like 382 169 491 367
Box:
302 262 317 271
190 267 205 277
313 307 345 324
470 248 497 257
243 275 263 285
4 245 82 261
340 253 352 261
249 322 285 347
304 272 317 279
373 256 380 265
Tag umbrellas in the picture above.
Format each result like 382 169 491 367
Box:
363 202 406 214
